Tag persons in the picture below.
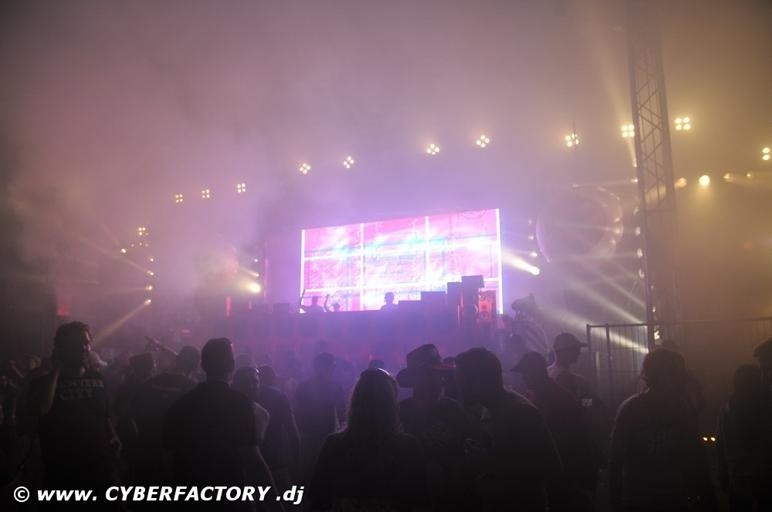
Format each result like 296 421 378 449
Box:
0 287 772 512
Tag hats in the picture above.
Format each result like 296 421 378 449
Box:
553 330 590 353
510 351 549 372
396 343 452 388
